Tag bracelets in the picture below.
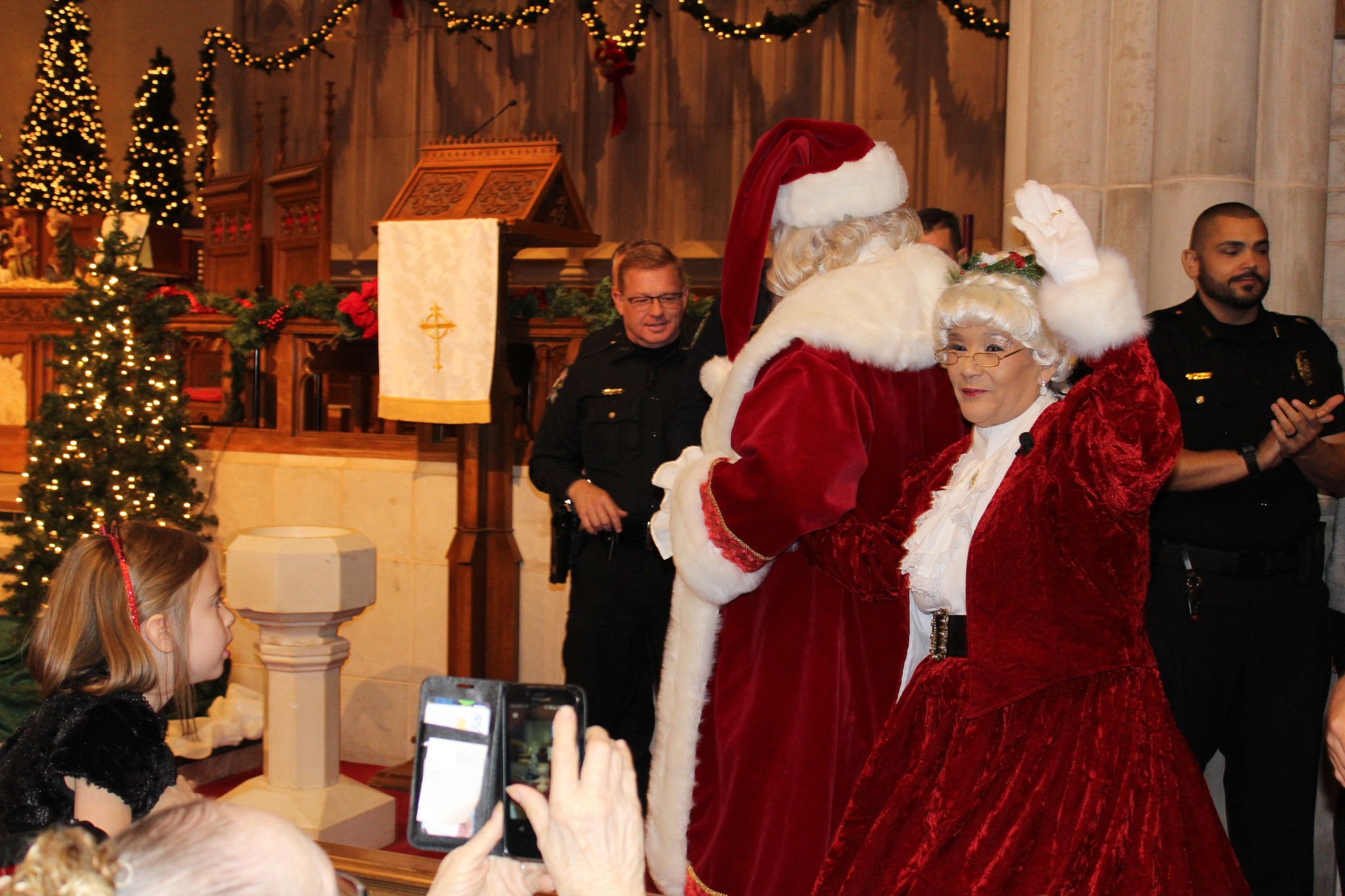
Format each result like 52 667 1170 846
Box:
1237 443 1261 480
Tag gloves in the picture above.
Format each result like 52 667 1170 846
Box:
1011 178 1099 282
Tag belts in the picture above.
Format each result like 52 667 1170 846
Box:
925 612 969 658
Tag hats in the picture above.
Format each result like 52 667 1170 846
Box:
696 117 908 399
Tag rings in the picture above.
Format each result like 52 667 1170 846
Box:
1051 210 1063 217
1285 429 1298 438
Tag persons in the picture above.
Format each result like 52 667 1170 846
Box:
913 207 968 269
0 517 235 870
818 181 1254 896
46 206 74 275
564 239 725 362
0 206 33 277
1071 196 1345 895
530 246 728 799
645 117 975 894
0 705 647 896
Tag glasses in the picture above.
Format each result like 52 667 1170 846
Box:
618 289 686 309
934 342 1027 369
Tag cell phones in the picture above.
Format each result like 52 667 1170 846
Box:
500 683 588 862
